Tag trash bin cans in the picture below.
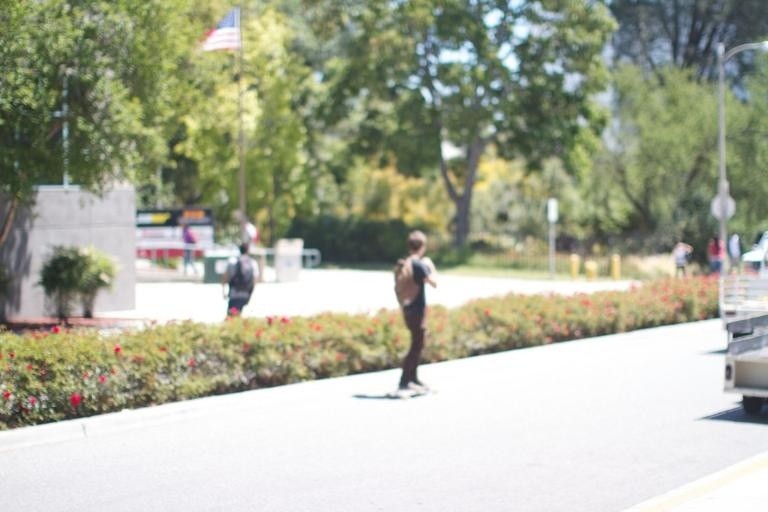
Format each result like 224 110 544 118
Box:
204 257 227 283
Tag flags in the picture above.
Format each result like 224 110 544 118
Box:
201 7 241 49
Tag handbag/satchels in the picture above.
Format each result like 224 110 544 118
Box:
394 257 420 308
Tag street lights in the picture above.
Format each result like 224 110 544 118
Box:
712 36 767 322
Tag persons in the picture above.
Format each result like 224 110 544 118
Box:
394 229 439 397
223 242 258 318
706 231 724 272
179 218 201 276
671 239 694 275
727 232 743 276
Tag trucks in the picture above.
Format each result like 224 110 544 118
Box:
711 228 767 420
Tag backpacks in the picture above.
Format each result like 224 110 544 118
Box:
230 257 255 292
